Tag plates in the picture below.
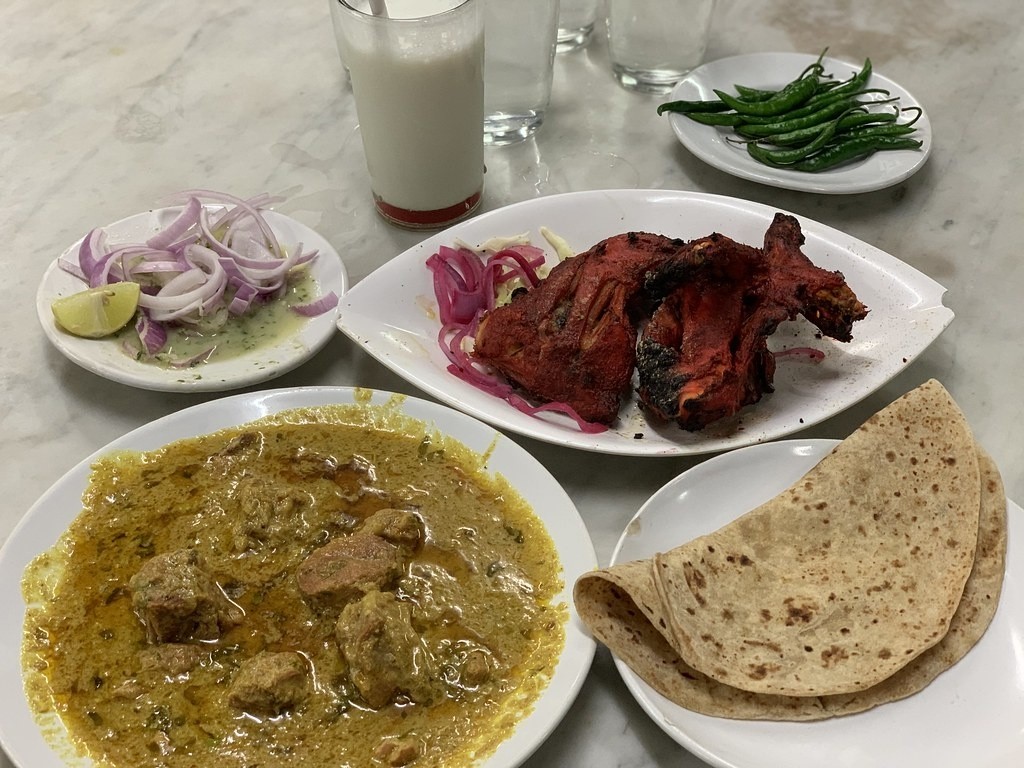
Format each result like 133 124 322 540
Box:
39 200 351 394
603 438 1023 767
670 53 935 194
1 382 603 767
338 188 948 458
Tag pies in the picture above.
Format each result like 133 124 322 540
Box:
573 378 1008 723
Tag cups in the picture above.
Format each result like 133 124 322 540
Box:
475 0 557 147
604 1 717 96
552 0 601 57
330 1 486 228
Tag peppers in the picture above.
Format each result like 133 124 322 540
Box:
658 45 923 171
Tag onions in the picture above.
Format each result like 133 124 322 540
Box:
58 191 339 367
